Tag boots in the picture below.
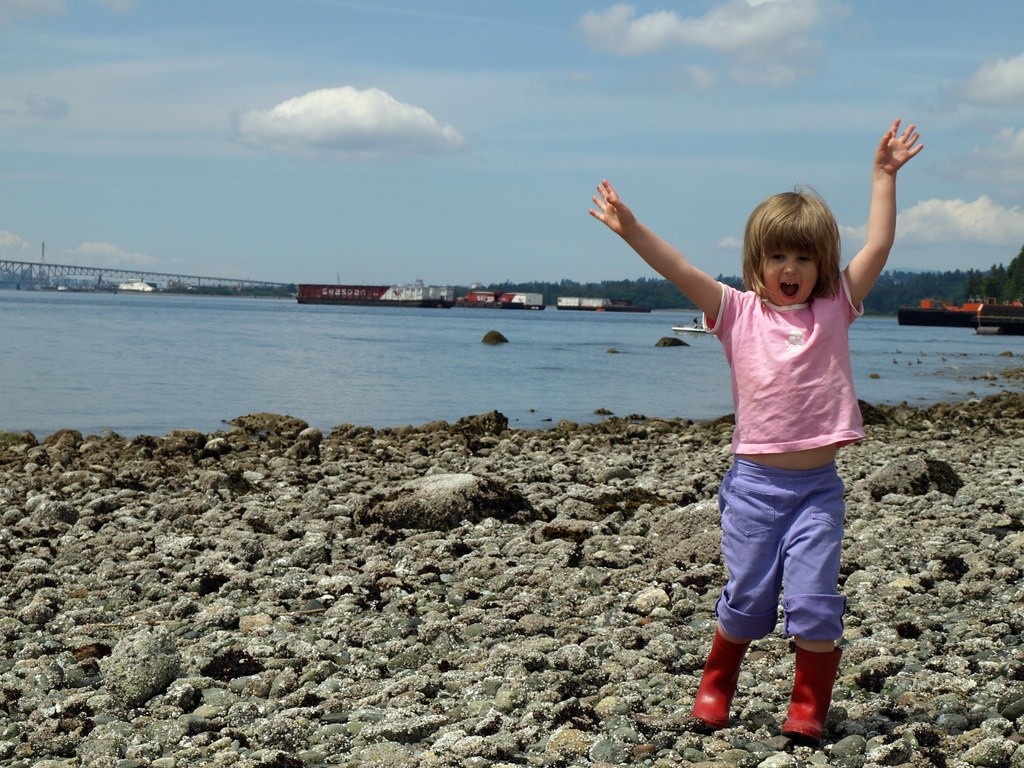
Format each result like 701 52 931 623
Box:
691 627 752 730
782 646 843 747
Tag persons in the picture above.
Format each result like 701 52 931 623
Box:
588 119 925 739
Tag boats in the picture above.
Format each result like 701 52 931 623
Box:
898 294 1024 335
458 301 547 311
672 313 707 335
295 297 456 310
554 305 653 313
